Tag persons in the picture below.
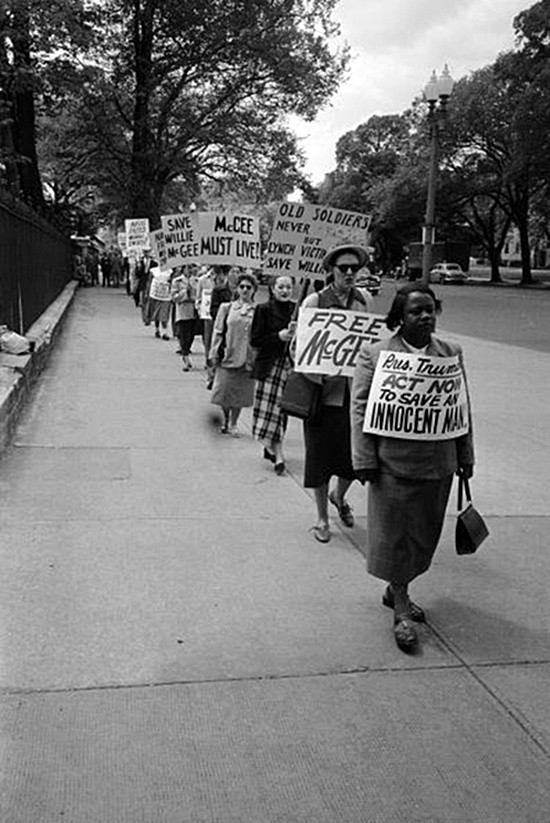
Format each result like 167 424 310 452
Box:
75 249 228 371
250 277 299 475
290 245 374 542
209 275 260 436
350 284 473 646
210 267 241 320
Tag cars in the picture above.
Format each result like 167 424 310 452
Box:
431 260 466 285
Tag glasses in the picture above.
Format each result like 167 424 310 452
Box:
238 285 253 290
331 260 360 273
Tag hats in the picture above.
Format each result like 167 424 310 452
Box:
323 244 369 272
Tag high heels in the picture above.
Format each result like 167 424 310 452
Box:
264 447 277 462
274 461 284 475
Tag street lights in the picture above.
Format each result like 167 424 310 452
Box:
419 63 453 284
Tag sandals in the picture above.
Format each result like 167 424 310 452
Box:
382 586 426 621
330 489 355 526
309 514 329 542
394 604 417 649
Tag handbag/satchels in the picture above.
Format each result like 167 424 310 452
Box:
455 472 489 554
276 373 320 422
217 340 227 362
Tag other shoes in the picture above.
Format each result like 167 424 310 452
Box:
230 426 240 437
221 423 228 431
155 331 160 338
162 335 171 341
